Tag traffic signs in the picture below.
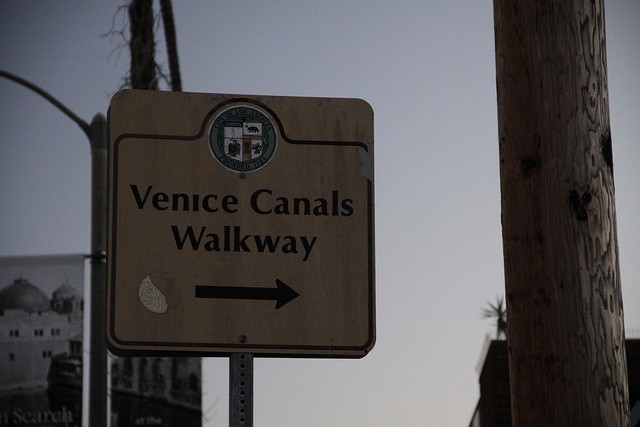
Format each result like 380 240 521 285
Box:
101 89 376 359
